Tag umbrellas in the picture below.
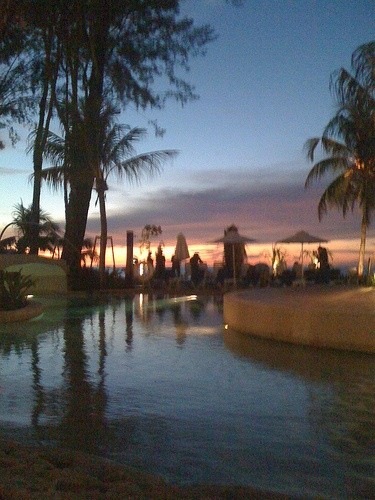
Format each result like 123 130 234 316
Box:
276 228 330 287
174 231 190 280
213 230 257 289
